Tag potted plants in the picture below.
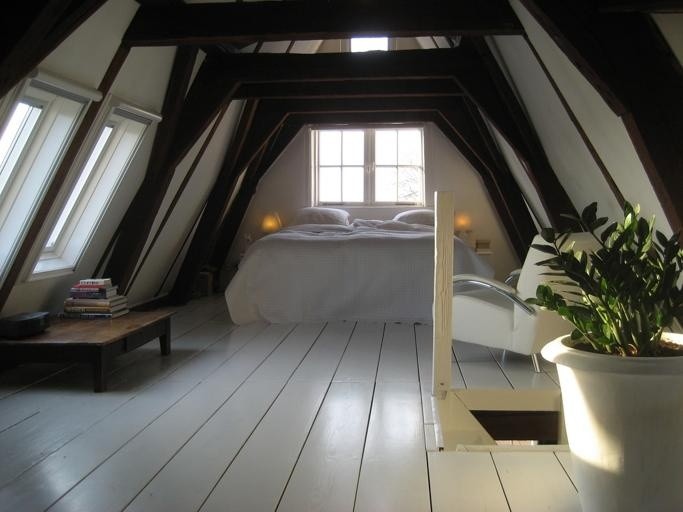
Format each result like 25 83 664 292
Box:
522 200 683 512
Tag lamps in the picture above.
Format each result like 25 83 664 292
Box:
452 209 473 248
260 211 281 234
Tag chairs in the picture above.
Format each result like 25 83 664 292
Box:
451 231 603 374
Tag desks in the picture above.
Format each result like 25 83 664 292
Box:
1 309 179 393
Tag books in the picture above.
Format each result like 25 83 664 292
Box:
55 279 130 318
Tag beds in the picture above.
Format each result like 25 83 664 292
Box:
225 223 484 325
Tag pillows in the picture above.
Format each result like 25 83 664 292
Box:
392 209 434 225
289 206 350 226
